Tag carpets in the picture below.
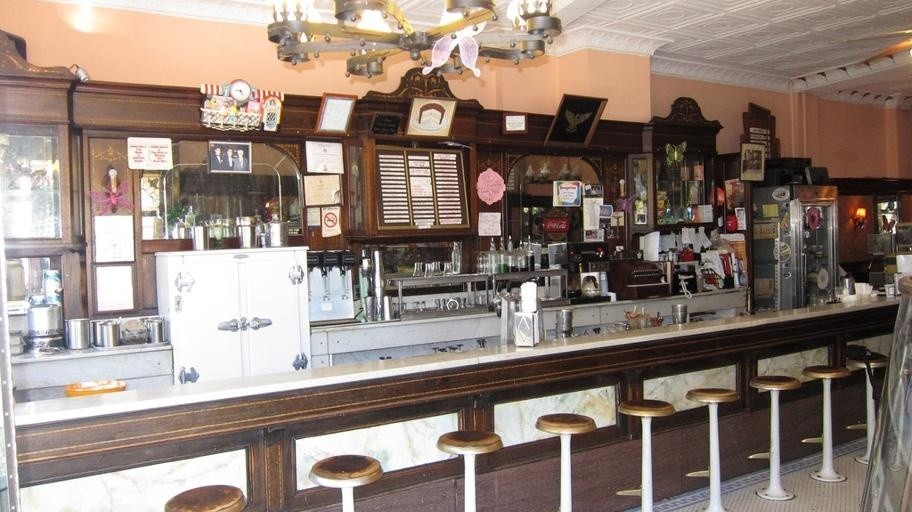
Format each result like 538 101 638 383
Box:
625 435 870 512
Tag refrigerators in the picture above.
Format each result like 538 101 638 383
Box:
750 182 840 307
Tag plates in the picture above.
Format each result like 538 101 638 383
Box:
64 379 127 398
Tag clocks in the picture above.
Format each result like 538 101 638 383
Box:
228 79 251 103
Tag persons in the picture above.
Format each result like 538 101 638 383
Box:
103 169 123 213
212 145 227 170
222 147 236 170
233 149 249 171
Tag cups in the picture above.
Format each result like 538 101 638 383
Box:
357 249 393 324
555 309 574 338
883 283 896 299
235 216 255 249
144 318 163 344
672 305 688 323
190 225 205 250
411 260 453 278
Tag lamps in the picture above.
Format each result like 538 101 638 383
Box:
266 0 562 78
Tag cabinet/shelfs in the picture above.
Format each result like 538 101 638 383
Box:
152 245 312 387
347 135 474 243
627 152 718 238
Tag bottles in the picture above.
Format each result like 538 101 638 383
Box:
476 228 535 274
263 213 290 246
451 240 461 274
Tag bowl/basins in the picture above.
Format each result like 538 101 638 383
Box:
119 328 151 346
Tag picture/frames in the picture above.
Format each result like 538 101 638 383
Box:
502 111 530 135
315 92 357 135
544 94 609 148
740 140 766 181
403 96 458 139
207 139 252 176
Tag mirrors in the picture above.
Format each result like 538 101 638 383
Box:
136 138 306 240
505 151 605 255
